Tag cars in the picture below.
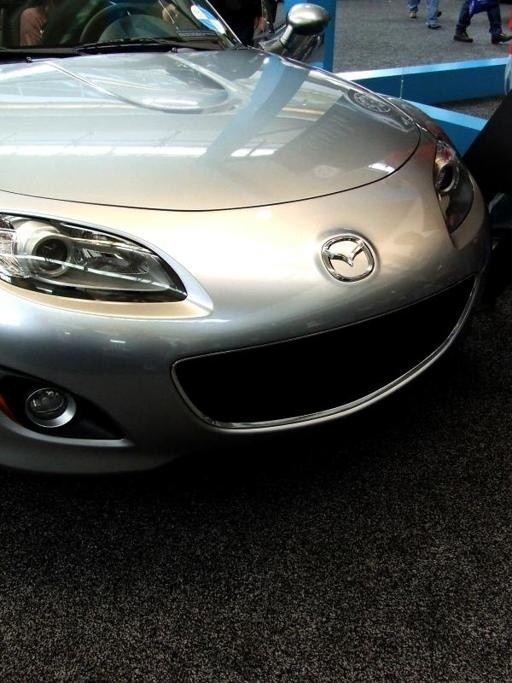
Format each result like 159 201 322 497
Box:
1 0 496 476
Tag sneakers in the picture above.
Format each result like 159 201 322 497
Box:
408 10 512 43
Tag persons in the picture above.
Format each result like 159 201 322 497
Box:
407 0 442 29
454 0 512 44
